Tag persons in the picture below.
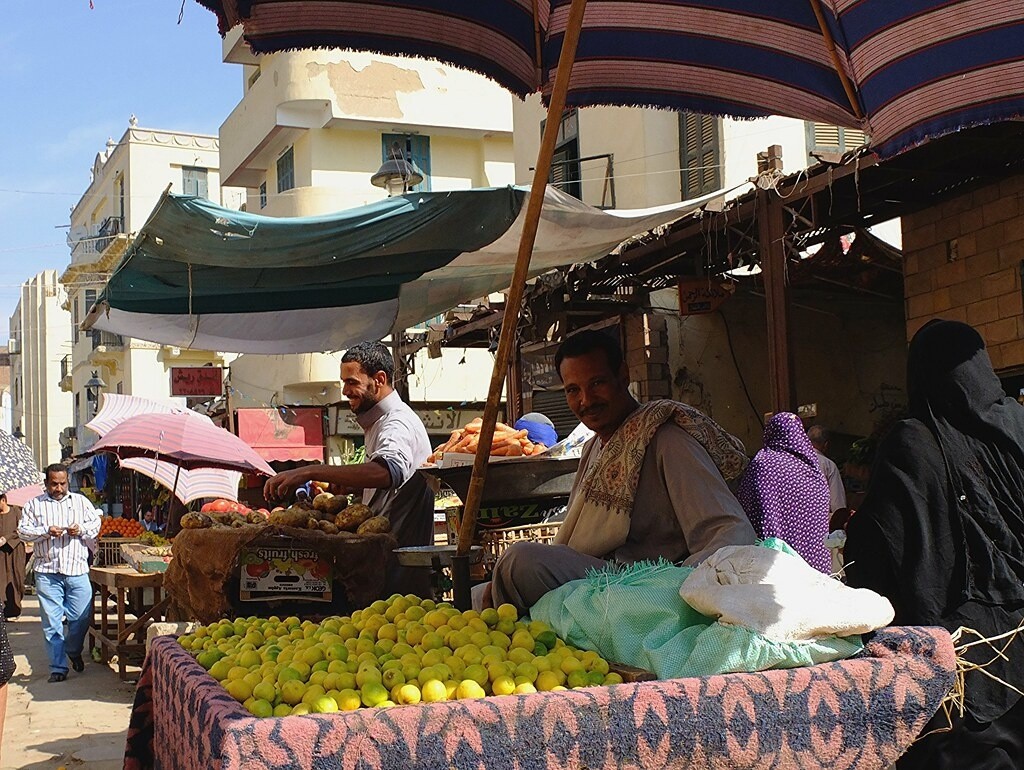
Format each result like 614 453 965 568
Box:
17 463 102 683
294 486 308 501
264 341 435 612
482 329 756 626
737 412 883 579
141 510 160 534
0 494 28 764
845 318 1024 770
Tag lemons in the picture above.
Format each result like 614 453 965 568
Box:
136 531 170 547
177 593 624 719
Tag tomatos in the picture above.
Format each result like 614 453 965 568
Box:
201 499 284 519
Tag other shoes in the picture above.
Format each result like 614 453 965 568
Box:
48 672 68 683
66 652 85 673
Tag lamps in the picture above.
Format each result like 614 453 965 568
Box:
83 369 108 412
13 426 26 443
370 143 423 197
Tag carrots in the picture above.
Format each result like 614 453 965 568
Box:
425 418 547 463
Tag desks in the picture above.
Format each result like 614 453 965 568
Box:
90 564 165 681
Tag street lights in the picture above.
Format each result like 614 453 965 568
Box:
84 369 112 517
370 142 424 404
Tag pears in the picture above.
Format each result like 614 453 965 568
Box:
142 547 173 557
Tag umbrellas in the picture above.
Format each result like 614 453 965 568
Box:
81 391 279 506
193 0 1024 158
0 428 40 495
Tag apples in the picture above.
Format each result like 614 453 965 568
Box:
99 515 145 538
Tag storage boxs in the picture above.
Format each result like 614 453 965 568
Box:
241 542 333 603
